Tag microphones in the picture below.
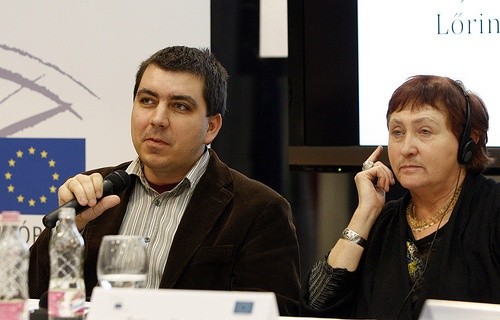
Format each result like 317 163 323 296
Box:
43 170 129 229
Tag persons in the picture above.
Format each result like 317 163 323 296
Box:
303 75 500 320
28 46 303 317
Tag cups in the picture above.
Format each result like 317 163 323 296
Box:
96 235 150 290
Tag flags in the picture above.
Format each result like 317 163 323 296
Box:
0 137 86 216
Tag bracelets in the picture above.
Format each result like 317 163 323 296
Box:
339 227 368 248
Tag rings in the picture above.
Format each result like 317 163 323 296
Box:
361 161 373 170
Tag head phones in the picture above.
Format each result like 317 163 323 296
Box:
454 81 475 165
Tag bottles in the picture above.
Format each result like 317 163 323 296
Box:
0 210 31 320
47 208 86 320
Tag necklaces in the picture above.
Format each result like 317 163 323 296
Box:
404 180 462 233
